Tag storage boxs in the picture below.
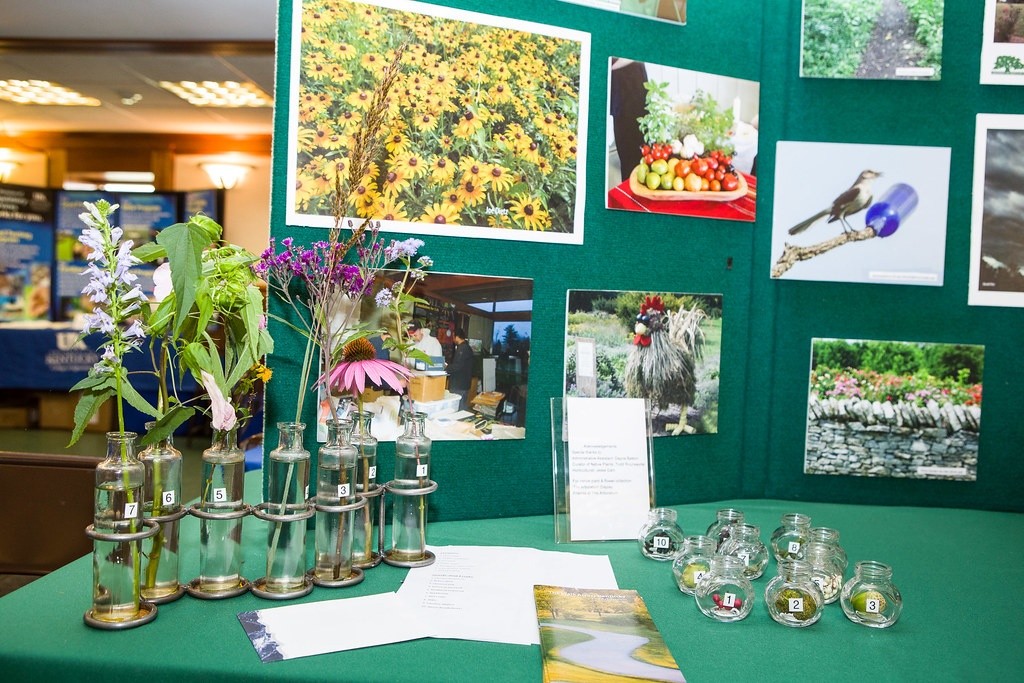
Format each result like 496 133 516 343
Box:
413 356 445 372
0 407 41 427
41 388 115 433
407 372 451 403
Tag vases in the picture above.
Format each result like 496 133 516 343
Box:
313 419 357 583
337 408 381 562
90 431 141 622
197 419 242 592
134 420 181 597
264 421 311 594
391 412 430 561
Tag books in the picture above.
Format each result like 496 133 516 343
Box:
533 584 686 683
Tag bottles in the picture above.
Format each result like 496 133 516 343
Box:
720 522 770 578
839 561 904 629
708 508 743 553
765 512 849 627
695 556 755 622
637 508 684 561
672 534 717 594
865 182 919 239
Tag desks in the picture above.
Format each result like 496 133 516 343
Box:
0 468 1024 683
608 170 756 221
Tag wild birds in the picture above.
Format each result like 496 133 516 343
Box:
788 169 883 240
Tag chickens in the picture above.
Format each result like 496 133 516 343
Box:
626 295 702 436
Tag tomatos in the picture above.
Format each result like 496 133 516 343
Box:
636 144 739 192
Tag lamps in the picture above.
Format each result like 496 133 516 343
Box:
197 162 256 190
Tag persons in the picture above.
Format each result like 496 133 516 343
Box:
407 320 443 369
0 274 24 320
29 266 49 316
445 328 475 411
365 331 390 398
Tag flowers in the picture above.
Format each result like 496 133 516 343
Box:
373 237 435 554
250 224 383 581
64 199 274 615
308 331 415 558
